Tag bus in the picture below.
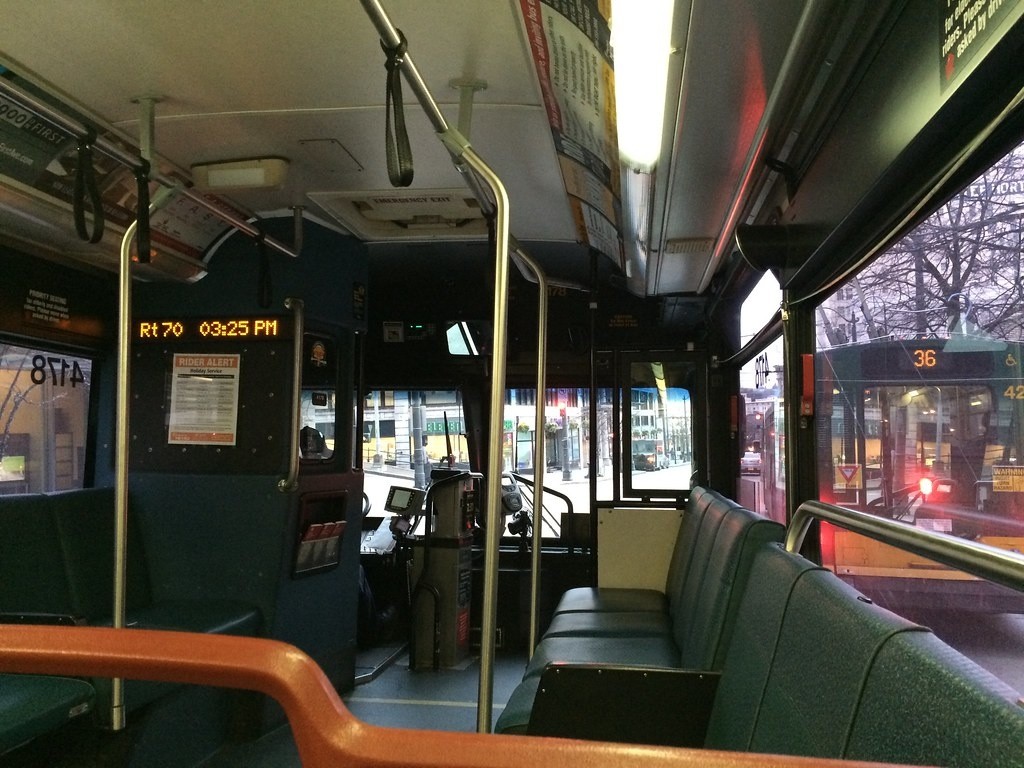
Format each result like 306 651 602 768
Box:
762 328 1024 621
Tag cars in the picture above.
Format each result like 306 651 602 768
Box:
741 452 762 475
656 453 670 471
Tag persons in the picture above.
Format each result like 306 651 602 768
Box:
421 434 434 486
668 447 686 460
298 425 334 460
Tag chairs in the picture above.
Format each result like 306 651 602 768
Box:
0 492 175 729
497 481 1024 768
43 485 262 635
0 672 101 768
